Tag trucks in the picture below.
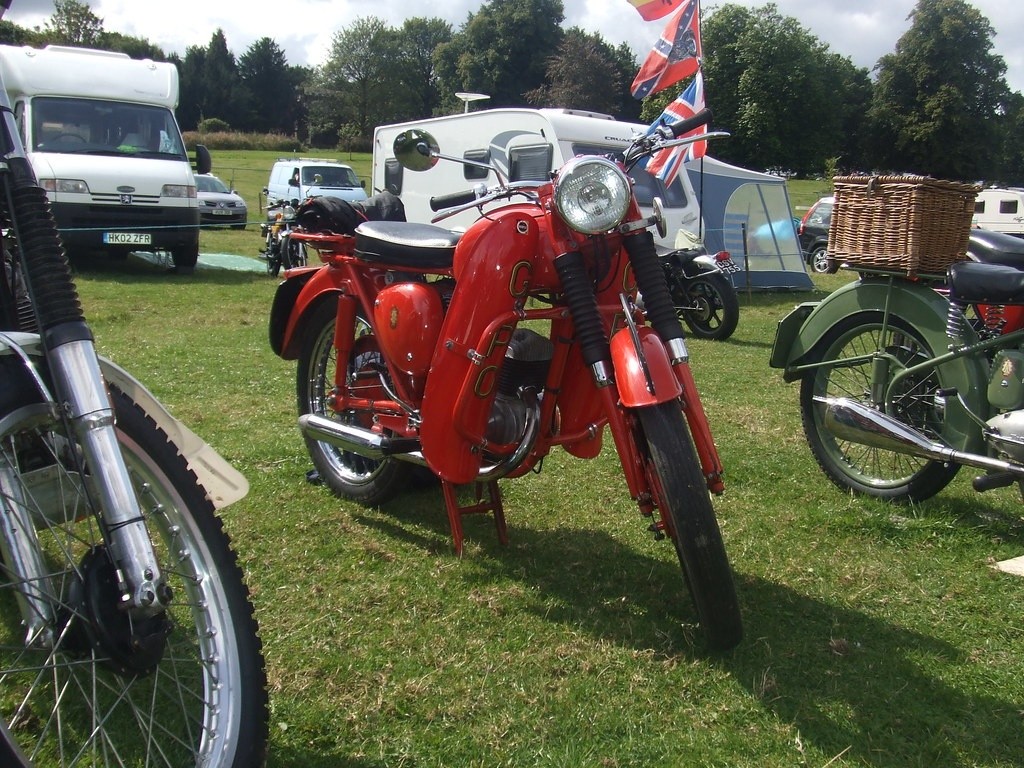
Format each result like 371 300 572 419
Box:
0 44 214 273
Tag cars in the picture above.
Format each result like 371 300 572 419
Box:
798 196 843 275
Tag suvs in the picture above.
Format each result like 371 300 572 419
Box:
193 173 249 230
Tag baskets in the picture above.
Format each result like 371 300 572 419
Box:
826 175 983 272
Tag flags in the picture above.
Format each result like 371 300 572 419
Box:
623 69 709 191
626 0 701 22
630 1 702 101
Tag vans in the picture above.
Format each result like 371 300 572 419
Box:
968 188 1024 236
267 156 369 214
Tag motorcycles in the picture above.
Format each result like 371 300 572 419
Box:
369 107 741 342
268 107 747 659
259 174 324 280
0 80 271 768
768 169 1024 510
